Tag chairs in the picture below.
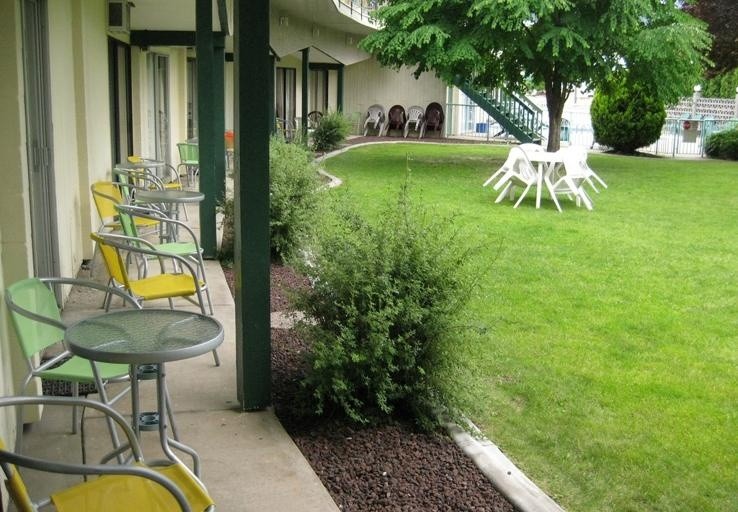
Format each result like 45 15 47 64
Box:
481 141 610 215
1 275 182 466
0 395 216 512
115 205 215 315
362 102 444 139
88 230 223 367
274 109 325 145
86 133 234 265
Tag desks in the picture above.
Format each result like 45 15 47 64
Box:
59 306 228 482
